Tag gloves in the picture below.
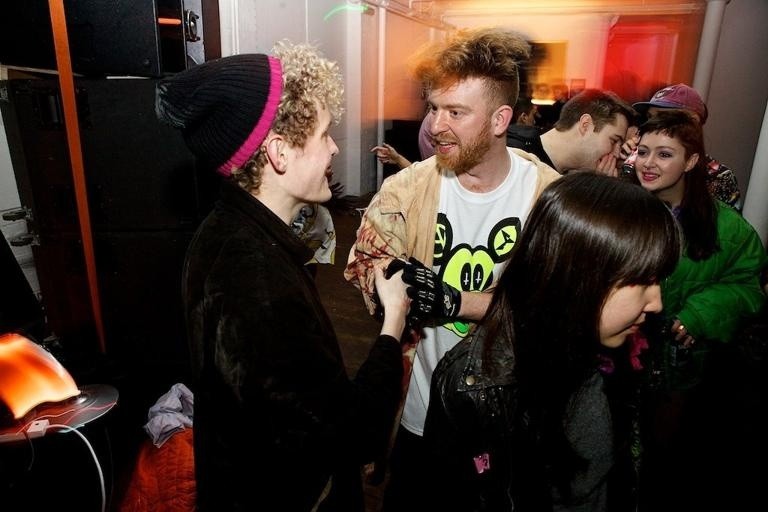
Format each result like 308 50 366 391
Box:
373 257 460 330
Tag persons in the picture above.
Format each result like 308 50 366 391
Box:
630 109 767 406
506 87 639 179
343 27 562 512
368 135 412 170
506 101 544 153
620 82 740 217
418 105 436 161
154 38 413 511
413 170 680 510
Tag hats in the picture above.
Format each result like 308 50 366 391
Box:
631 83 704 123
156 54 281 176
